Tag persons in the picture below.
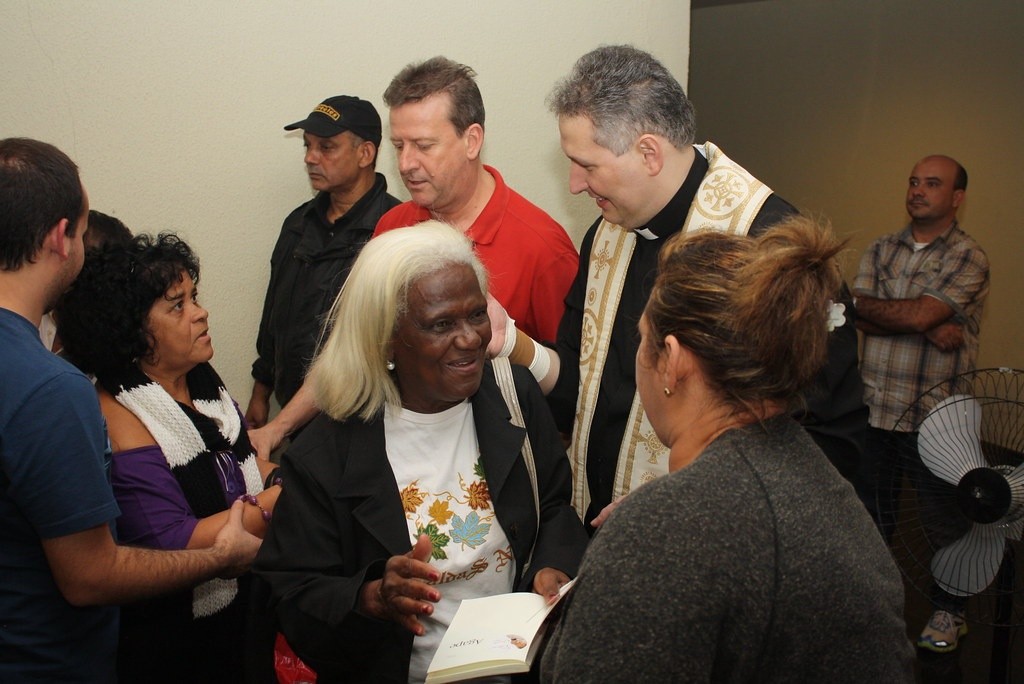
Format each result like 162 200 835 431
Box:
243 95 402 432
250 218 592 684
54 232 281 619
484 46 872 533
539 217 916 684
38 211 135 358
0 138 266 684
249 56 580 462
851 156 990 609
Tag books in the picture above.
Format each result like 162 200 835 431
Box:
425 575 579 684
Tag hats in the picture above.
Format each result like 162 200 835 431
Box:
283 95 383 147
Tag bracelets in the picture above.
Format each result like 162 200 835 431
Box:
273 478 283 485
240 494 271 524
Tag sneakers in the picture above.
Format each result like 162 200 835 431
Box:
916 609 968 652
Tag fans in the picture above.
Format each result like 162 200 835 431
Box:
874 368 1024 684
